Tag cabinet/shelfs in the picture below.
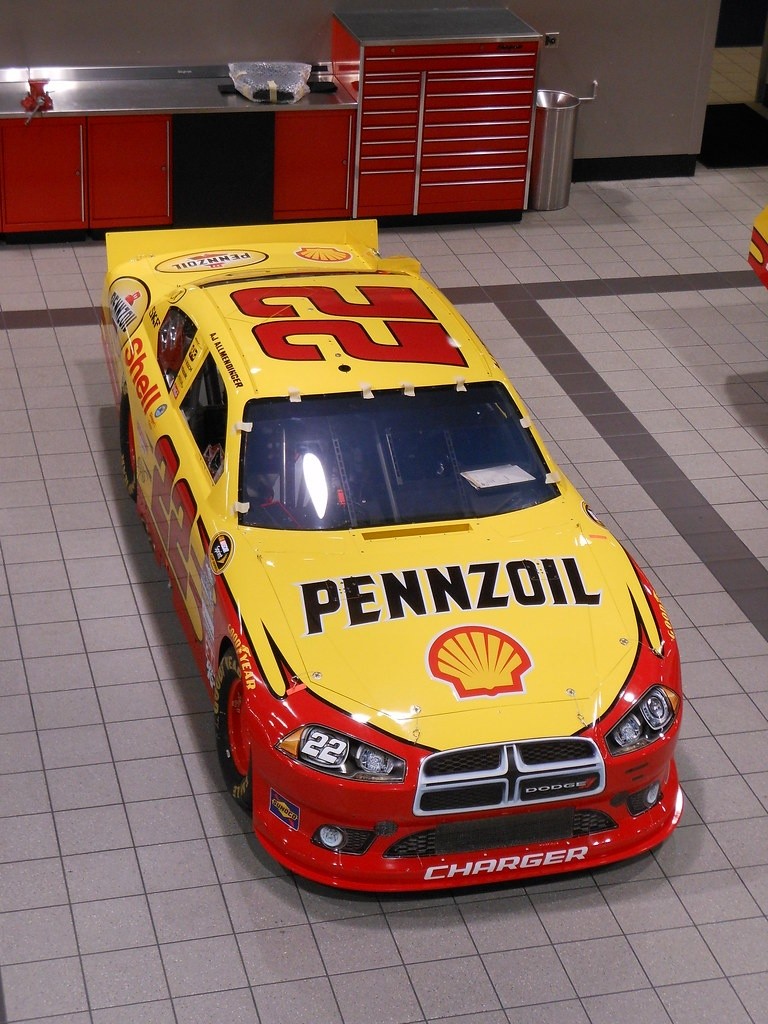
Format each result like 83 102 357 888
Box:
87 109 357 242
330 15 543 228
0 116 89 246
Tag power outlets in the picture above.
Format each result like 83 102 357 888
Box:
546 32 560 48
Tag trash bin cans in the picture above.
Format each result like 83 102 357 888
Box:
526 89 581 211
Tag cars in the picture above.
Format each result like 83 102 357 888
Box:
104 217 684 899
